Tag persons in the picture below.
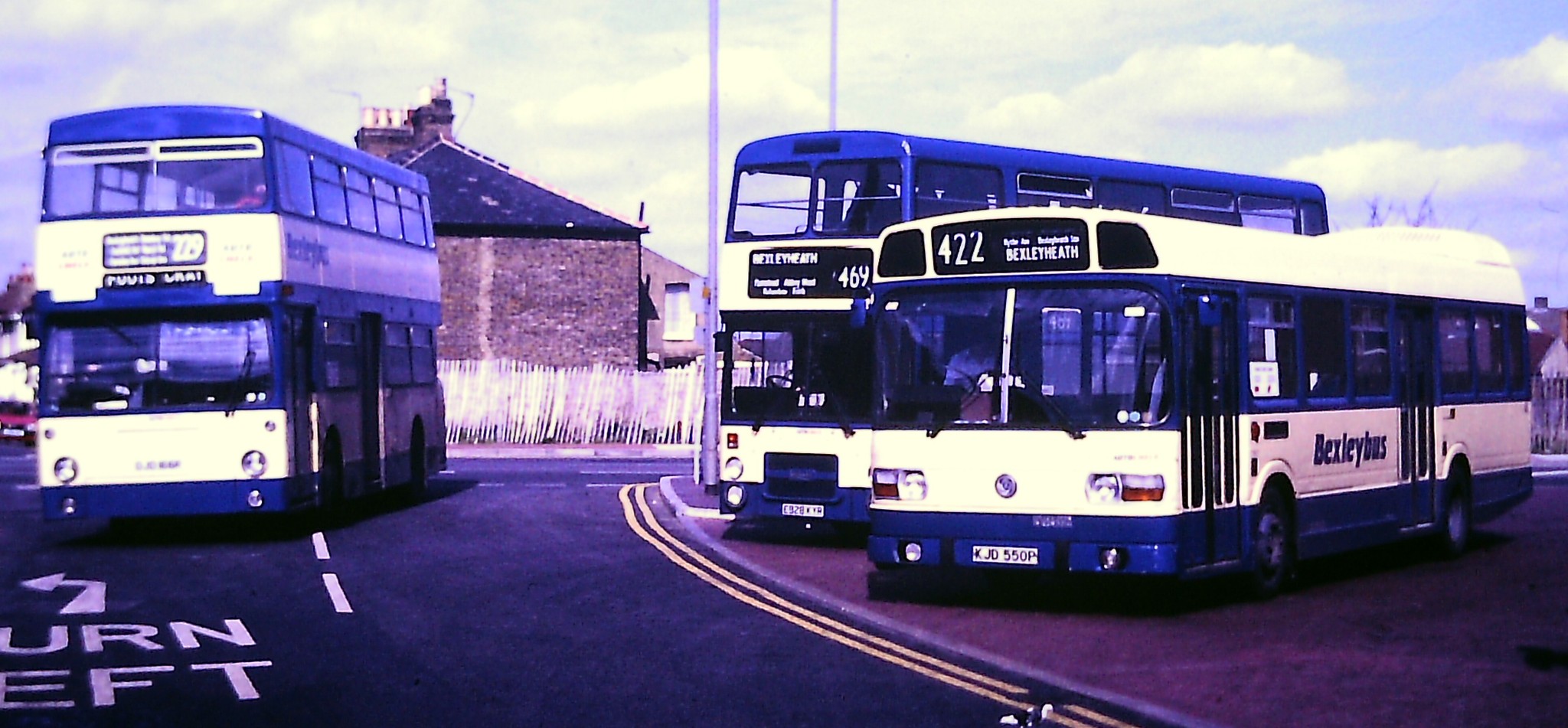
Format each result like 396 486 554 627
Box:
940 308 1005 394
231 172 294 212
830 162 1228 244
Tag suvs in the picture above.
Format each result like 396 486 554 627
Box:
0 399 38 446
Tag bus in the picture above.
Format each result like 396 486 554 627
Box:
715 127 1331 560
25 101 447 527
848 203 1539 605
